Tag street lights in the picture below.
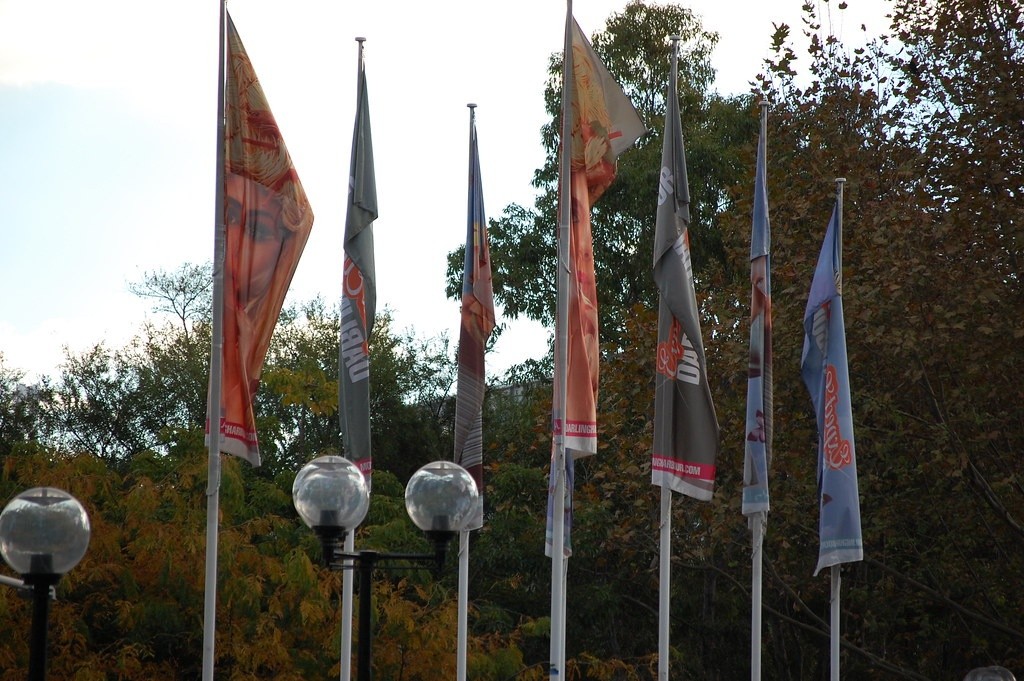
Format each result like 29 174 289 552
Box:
292 456 479 681
1 485 92 681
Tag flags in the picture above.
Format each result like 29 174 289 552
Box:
546 14 648 562
800 198 865 579
650 53 722 503
337 65 379 494
454 117 496 533
741 133 774 535
207 8 316 467
